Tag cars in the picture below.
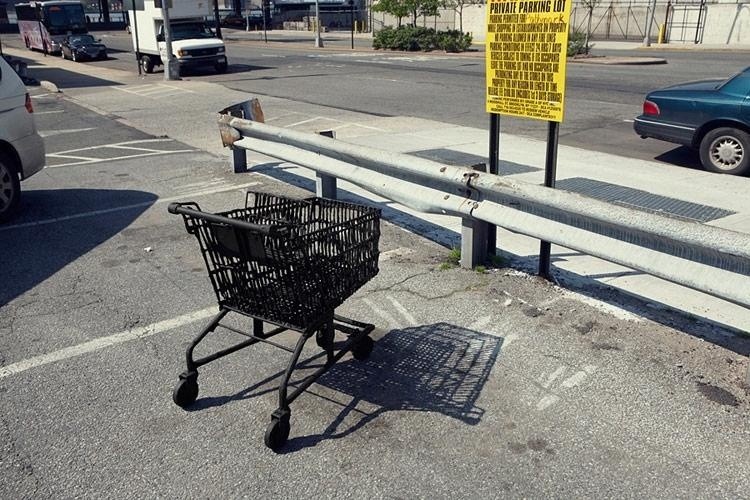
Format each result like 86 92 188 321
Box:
0 51 46 214
126 25 131 34
633 66 749 174
59 34 108 62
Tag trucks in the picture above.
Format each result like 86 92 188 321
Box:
128 0 227 74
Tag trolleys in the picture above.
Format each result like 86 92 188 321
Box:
168 191 381 450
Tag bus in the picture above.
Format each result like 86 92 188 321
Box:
14 1 87 56
